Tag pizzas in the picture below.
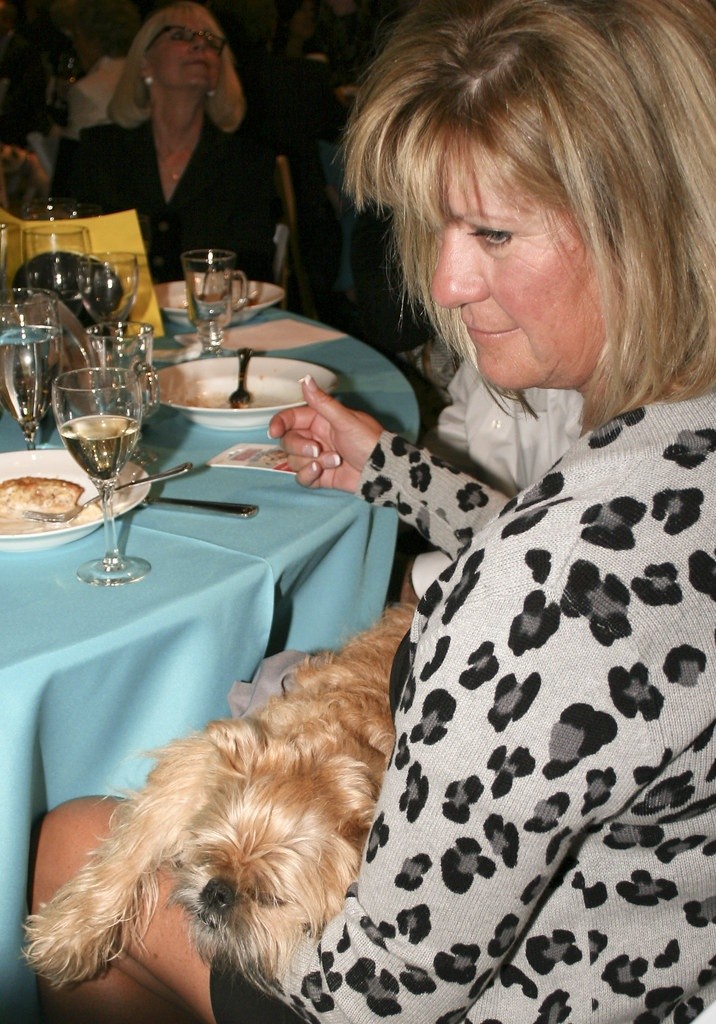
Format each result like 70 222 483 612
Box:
0 476 85 516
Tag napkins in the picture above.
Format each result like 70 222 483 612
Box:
175 316 348 355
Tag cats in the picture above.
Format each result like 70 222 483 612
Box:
0 144 50 214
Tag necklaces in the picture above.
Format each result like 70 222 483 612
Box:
154 130 197 184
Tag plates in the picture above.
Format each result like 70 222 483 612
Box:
0 450 151 552
152 357 337 431
156 280 285 326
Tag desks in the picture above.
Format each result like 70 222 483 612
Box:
0 306 420 1024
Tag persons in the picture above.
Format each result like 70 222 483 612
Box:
24 0 716 1024
0 0 588 495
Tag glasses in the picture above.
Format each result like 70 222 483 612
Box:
144 25 226 58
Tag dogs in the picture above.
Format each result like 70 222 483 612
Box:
20 603 413 991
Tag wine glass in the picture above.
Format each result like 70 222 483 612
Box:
52 368 151 586
0 198 248 467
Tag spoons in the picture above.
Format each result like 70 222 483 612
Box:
229 348 251 409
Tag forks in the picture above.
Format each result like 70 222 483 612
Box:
23 461 194 525
